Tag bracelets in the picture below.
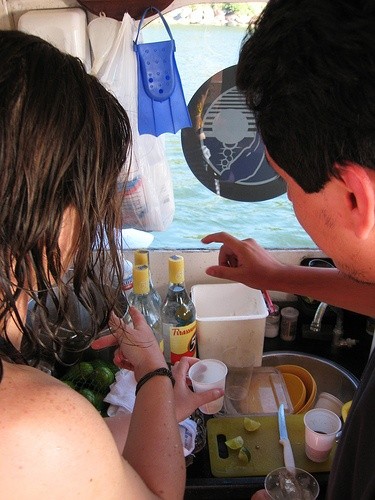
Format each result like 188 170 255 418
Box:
135 368 176 395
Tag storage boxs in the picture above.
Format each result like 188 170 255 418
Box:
190 283 269 367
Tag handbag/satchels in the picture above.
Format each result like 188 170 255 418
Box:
88 14 177 234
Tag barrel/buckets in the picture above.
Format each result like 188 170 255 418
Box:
25 268 131 365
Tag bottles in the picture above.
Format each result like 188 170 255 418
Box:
109 248 134 294
265 305 299 341
125 249 199 364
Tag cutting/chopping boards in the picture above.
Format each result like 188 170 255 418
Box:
206 413 340 477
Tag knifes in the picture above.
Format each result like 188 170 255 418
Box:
277 404 296 476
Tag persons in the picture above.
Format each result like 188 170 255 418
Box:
0 30 224 500
201 0 375 500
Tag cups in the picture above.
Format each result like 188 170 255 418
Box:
188 358 229 415
302 408 342 463
315 393 342 420
264 467 319 500
223 346 256 401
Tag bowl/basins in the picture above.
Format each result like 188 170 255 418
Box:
342 400 352 422
242 351 360 413
